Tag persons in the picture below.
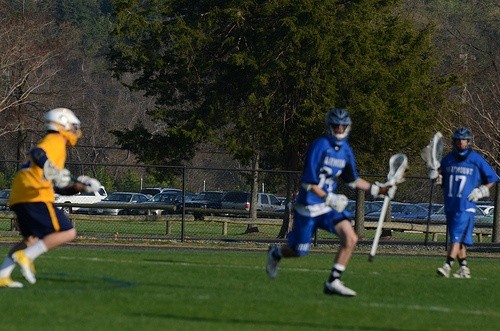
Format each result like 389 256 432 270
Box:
428 128 500 278
266 107 397 295
0 107 101 288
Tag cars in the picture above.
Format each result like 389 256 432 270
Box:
190 192 225 206
428 205 487 221
364 206 433 219
0 191 12 206
88 192 150 214
142 192 195 213
146 188 179 196
54 185 104 213
279 197 495 215
344 200 380 216
222 191 285 217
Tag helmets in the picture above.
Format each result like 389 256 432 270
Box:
44 108 82 148
451 128 472 157
326 108 352 145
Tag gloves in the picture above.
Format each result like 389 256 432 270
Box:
76 175 101 193
467 185 490 202
433 174 442 185
369 180 397 202
325 192 349 212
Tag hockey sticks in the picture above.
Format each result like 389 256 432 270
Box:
367 153 409 263
43 159 93 189
422 134 444 246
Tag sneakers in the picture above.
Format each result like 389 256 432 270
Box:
10 247 37 284
436 262 452 278
266 245 282 279
324 279 357 297
452 267 472 279
0 274 24 288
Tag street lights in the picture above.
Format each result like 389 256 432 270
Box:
9 60 27 183
